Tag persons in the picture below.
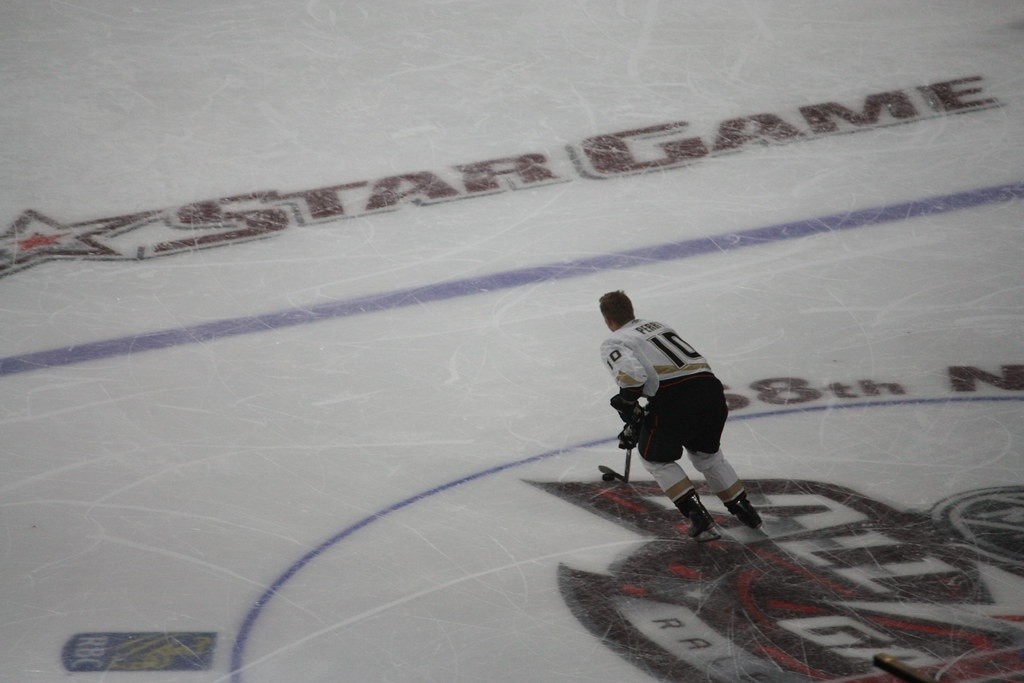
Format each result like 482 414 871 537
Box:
598 292 763 543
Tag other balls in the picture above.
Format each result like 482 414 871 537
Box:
601 473 615 481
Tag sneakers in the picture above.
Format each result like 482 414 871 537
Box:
728 496 768 536
675 493 722 543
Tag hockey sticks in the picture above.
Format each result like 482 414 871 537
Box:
598 449 632 484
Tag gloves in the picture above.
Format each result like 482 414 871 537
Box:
610 394 637 423
618 425 641 449
